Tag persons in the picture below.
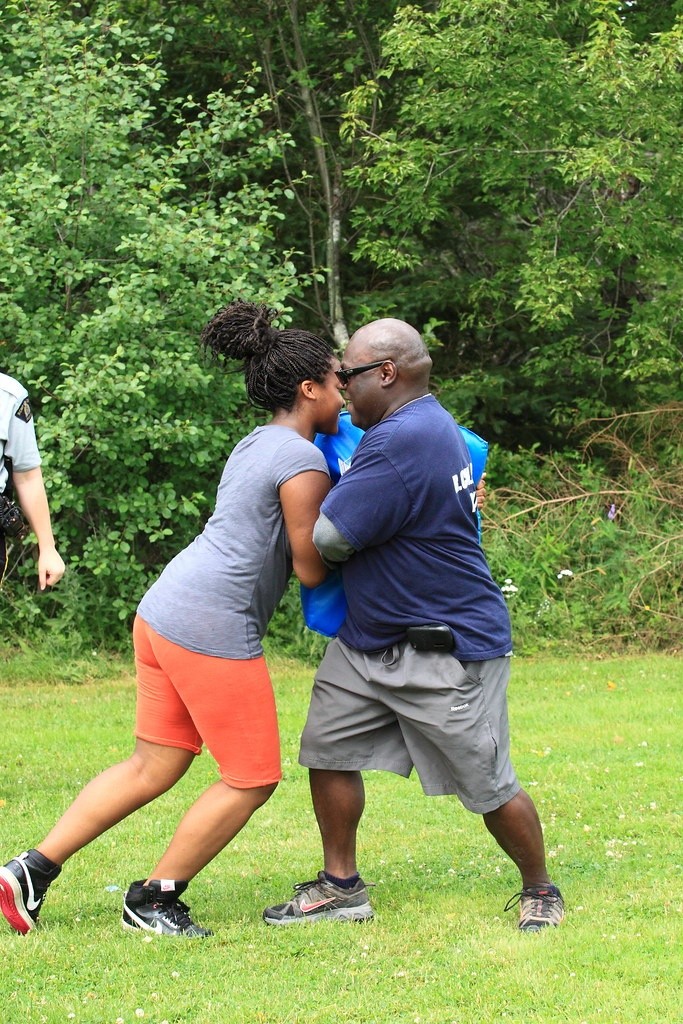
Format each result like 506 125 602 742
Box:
0 301 488 941
264 318 566 934
0 373 67 590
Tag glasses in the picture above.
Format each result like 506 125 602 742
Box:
335 362 383 387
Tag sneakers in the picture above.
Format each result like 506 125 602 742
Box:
518 885 564 928
0 850 63 932
120 879 215 937
263 874 374 927
